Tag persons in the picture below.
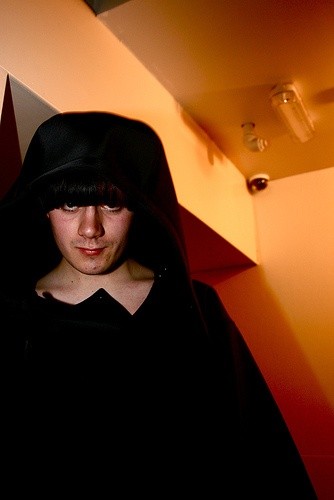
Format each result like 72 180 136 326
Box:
1 112 317 499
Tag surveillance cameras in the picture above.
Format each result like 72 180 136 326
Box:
248 173 271 192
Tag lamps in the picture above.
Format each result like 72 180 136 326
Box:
268 83 317 144
